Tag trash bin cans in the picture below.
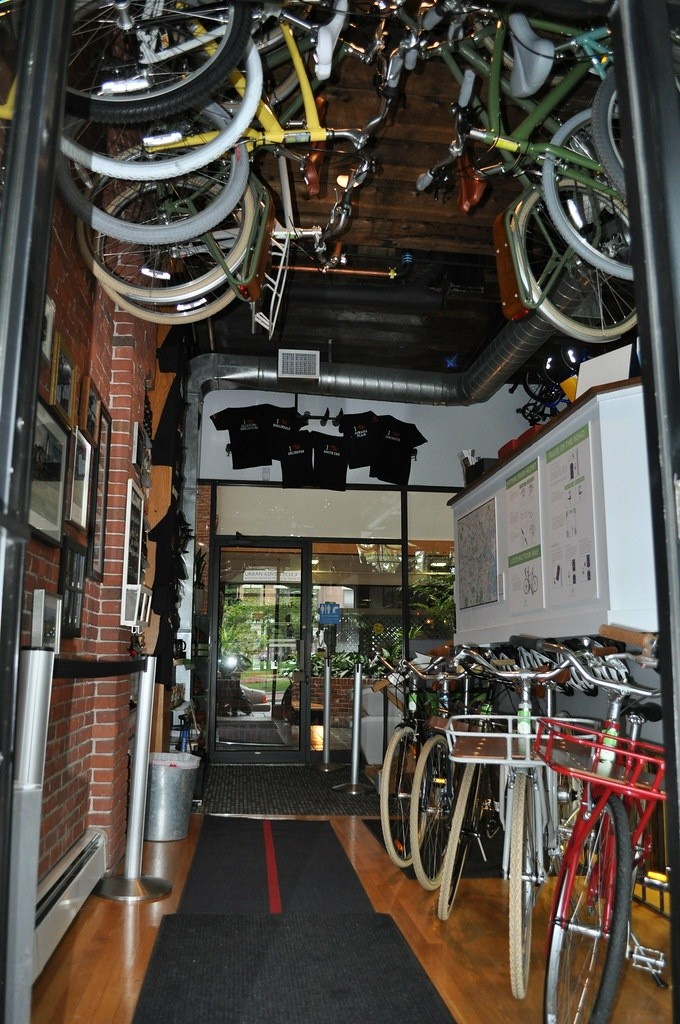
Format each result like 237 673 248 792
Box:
143 750 201 843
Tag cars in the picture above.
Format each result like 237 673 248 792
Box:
240 685 270 712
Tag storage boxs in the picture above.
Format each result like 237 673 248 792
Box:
466 425 543 486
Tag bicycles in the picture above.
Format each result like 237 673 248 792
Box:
391 635 497 891
508 344 589 427
363 644 451 871
44 0 640 346
511 635 679 1023
430 643 520 921
462 639 618 999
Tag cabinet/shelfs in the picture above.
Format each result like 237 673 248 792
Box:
447 376 660 645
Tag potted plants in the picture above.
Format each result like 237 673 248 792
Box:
219 628 234 717
194 549 208 615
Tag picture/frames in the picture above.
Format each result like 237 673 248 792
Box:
78 376 102 447
85 401 113 583
49 330 76 430
31 589 63 659
58 536 88 638
28 393 72 549
41 286 56 368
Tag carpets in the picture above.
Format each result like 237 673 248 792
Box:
130 763 589 1024
216 720 285 745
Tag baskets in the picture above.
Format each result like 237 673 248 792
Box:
532 717 668 803
442 707 600 767
